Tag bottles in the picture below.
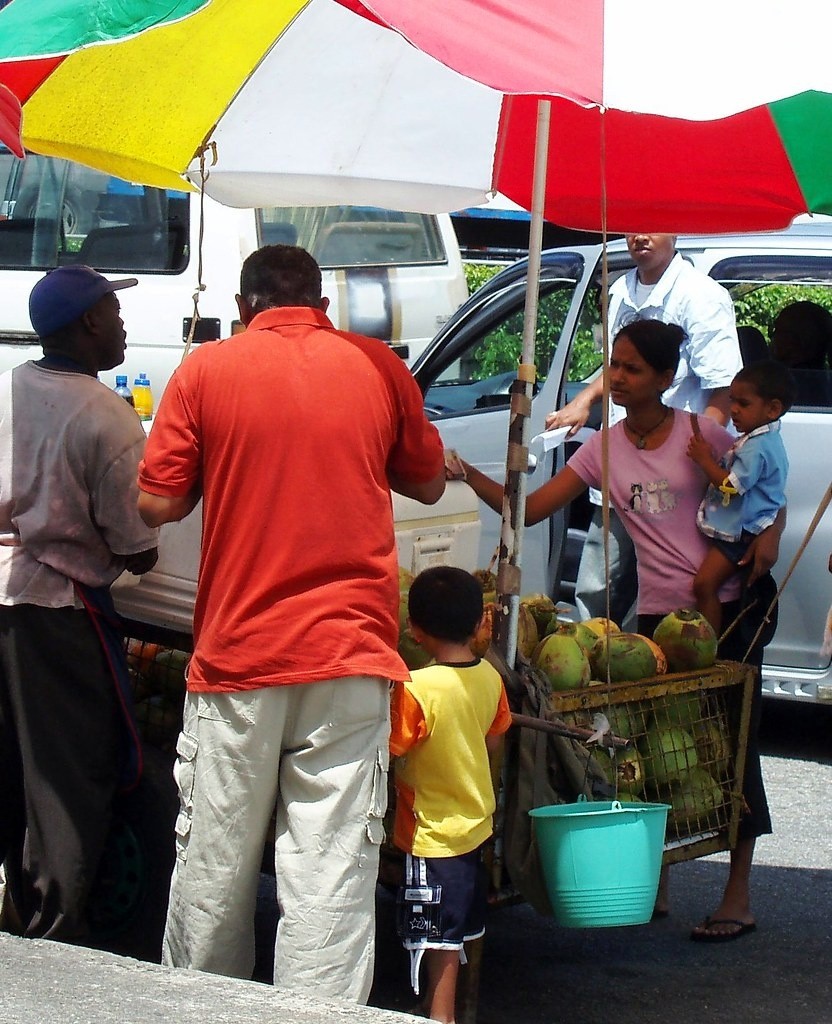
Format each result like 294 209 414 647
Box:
131 373 155 421
112 375 136 409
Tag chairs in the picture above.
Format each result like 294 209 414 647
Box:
0 218 58 265
311 222 423 264
768 301 832 407
737 326 767 367
76 219 186 270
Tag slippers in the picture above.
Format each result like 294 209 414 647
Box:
691 915 756 944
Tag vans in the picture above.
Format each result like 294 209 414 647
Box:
1 136 468 415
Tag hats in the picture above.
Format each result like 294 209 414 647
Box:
29 264 138 337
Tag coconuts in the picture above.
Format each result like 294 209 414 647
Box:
397 570 732 828
125 650 190 788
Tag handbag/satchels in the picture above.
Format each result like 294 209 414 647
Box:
741 574 778 648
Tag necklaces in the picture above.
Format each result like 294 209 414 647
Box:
621 405 673 448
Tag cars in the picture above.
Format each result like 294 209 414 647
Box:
397 221 831 718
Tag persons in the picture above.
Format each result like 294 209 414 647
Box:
446 229 789 946
135 243 445 1005
1 265 158 944
389 566 513 1023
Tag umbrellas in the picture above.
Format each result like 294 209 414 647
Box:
0 2 832 670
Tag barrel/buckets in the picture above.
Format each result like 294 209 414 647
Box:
527 800 672 930
